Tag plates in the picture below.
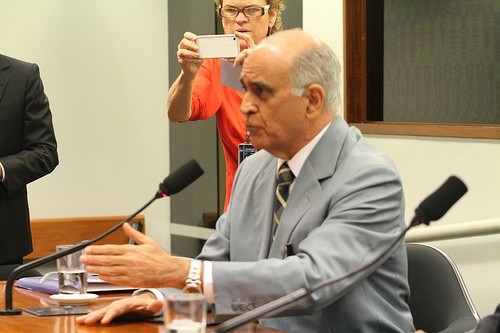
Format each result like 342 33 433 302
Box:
49 294 99 306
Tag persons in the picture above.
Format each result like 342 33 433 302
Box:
75 28 417 333
167 0 288 214
0 54 59 266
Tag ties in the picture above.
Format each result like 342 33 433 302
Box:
268 161 295 254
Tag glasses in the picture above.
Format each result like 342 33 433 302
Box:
218 3 271 18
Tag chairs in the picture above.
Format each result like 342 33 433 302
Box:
403 244 480 333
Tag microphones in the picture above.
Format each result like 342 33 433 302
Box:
0 158 205 316
210 175 468 333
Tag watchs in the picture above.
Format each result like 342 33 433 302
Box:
182 260 202 293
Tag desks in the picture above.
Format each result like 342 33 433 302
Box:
0 279 275 333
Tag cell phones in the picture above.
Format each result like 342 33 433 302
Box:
194 34 240 59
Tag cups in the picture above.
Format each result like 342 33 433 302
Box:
56 244 88 294
163 292 208 333
